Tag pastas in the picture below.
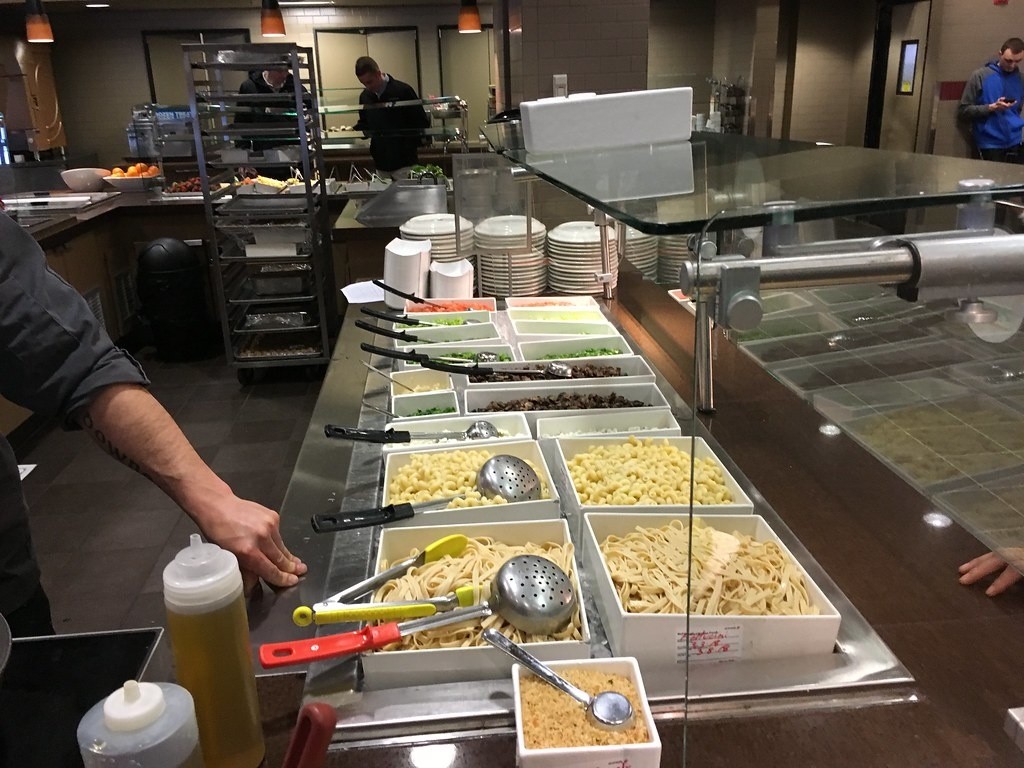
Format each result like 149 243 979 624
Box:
375 536 584 649
597 519 821 615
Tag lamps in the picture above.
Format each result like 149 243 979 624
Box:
24 0 55 43
260 0 286 38
458 0 482 34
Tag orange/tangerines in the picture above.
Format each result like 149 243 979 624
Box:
108 163 159 177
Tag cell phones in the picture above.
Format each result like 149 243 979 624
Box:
1003 98 1016 103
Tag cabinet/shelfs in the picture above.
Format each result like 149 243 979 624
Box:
179 42 336 387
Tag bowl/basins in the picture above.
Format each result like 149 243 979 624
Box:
60 168 112 192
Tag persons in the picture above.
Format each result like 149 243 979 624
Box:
0 201 310 639
234 52 316 183
959 544 1024 598
956 37 1024 236
345 56 433 180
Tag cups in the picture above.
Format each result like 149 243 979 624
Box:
14 155 26 163
691 111 721 133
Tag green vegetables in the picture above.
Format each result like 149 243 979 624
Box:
410 164 448 180
405 406 456 417
395 316 623 364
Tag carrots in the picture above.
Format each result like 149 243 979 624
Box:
408 300 494 312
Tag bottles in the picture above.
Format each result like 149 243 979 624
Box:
76 679 205 768
162 533 266 768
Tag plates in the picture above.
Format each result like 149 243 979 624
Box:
162 183 231 196
474 214 547 297
103 173 161 189
383 212 477 309
236 177 393 194
546 220 620 294
617 216 696 283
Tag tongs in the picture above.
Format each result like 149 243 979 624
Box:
348 164 364 183
371 172 387 185
291 534 488 627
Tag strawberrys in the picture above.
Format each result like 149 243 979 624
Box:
166 175 216 192
